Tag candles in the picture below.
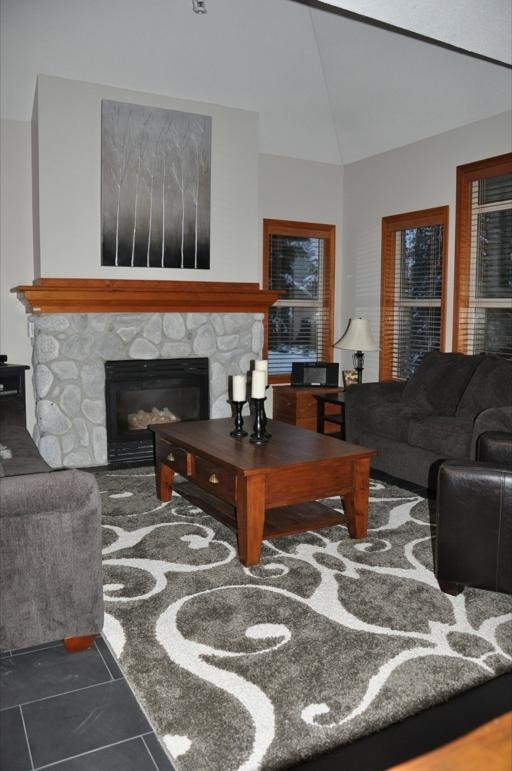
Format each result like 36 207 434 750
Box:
255 360 268 387
251 370 266 399
233 375 247 402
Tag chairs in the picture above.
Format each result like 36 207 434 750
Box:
428 431 512 596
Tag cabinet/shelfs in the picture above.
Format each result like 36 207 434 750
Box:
273 385 344 434
1 362 31 416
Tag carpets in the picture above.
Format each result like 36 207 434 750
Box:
71 459 512 771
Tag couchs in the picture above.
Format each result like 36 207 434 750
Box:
344 350 512 488
0 408 104 656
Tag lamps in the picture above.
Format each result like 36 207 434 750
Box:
333 318 382 384
